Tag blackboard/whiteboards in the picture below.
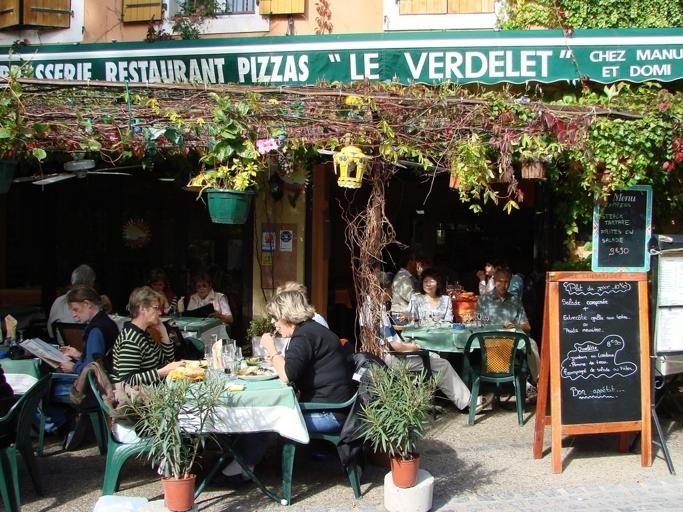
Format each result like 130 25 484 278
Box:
548 271 652 435
591 182 653 272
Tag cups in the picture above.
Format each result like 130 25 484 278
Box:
201 333 242 378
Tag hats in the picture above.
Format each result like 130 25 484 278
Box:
71 265 97 287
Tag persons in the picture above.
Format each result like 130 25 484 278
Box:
148 267 234 360
112 288 232 481
360 254 541 414
211 281 357 481
47 265 105 345
29 284 115 454
0 362 16 415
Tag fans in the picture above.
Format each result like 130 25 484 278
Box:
12 153 147 186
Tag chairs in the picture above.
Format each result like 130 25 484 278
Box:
281 336 372 507
89 371 171 491
127 376 217 512
1 320 101 512
354 312 528 427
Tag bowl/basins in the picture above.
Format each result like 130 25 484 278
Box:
6 345 24 360
244 357 259 366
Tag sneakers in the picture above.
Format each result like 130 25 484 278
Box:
474 393 495 414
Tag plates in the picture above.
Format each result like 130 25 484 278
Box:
168 358 209 383
238 366 278 380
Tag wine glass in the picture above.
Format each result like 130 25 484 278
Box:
177 303 184 319
17 329 26 342
415 310 489 329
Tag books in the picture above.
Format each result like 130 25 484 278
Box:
19 336 72 370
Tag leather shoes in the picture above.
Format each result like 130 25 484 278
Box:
210 471 253 488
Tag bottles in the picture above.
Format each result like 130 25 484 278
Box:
3 336 11 346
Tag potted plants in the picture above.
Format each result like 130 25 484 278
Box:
355 363 442 486
518 123 559 184
447 132 499 214
195 90 267 226
